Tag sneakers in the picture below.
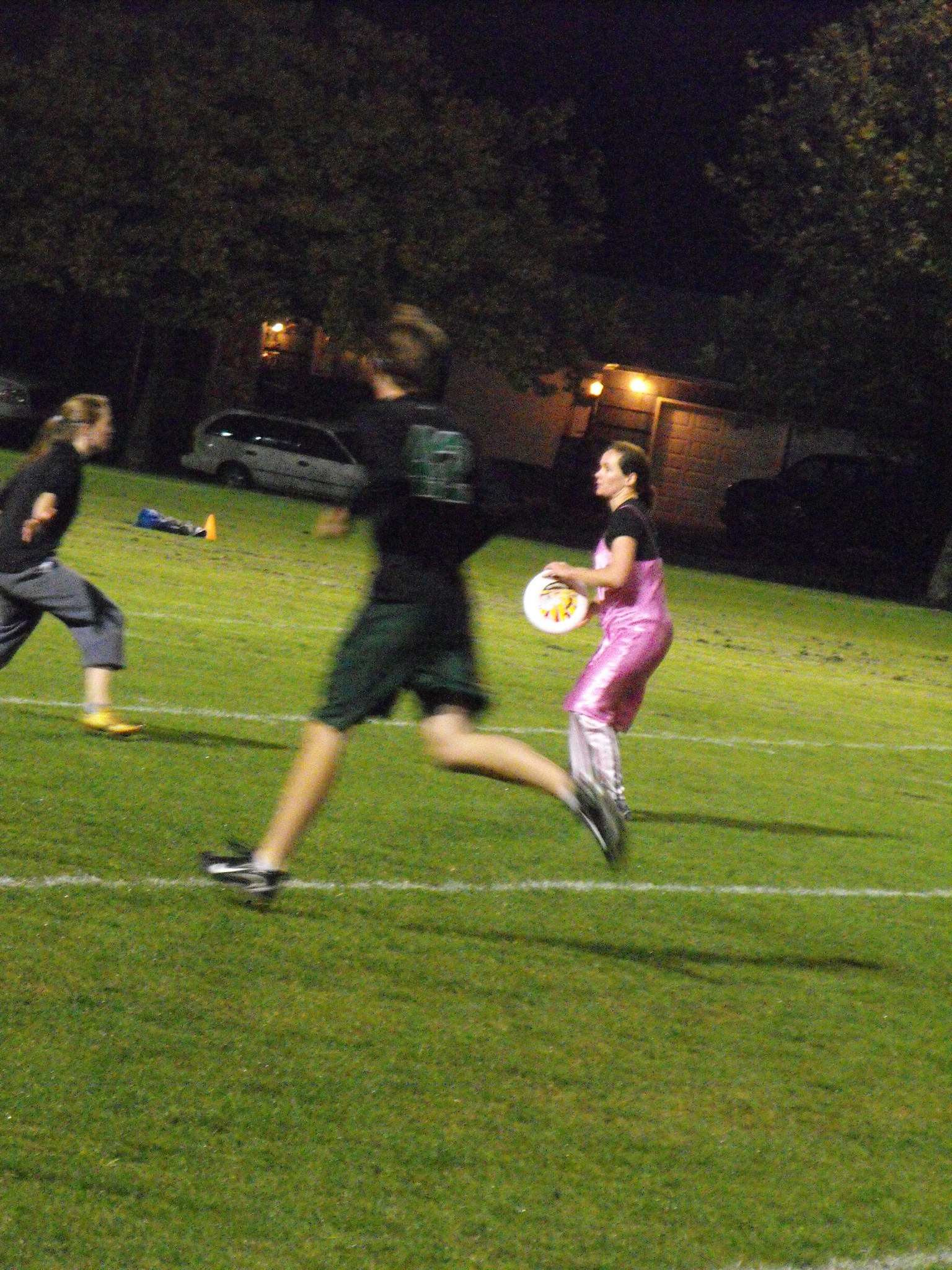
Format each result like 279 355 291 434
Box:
576 778 623 865
197 840 284 908
74 707 143 737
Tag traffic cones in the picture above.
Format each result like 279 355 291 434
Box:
204 513 217 540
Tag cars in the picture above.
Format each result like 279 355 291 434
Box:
179 408 374 505
0 375 35 445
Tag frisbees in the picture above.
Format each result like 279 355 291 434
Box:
523 568 591 635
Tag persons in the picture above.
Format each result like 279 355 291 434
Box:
200 303 628 903
0 395 148 740
546 437 674 817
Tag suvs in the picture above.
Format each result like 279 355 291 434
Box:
719 450 951 600
